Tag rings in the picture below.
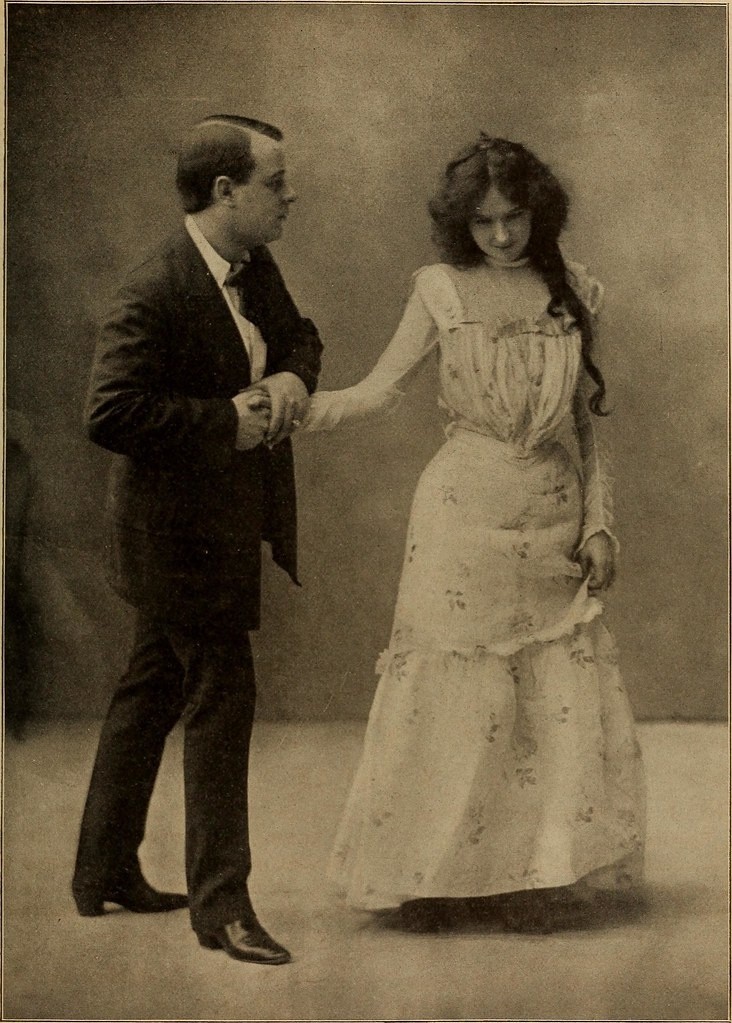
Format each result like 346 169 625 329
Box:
606 568 611 573
291 419 300 427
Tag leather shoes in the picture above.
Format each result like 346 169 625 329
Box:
196 916 292 965
72 875 188 916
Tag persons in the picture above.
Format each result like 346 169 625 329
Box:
68 116 322 964
258 127 646 925
